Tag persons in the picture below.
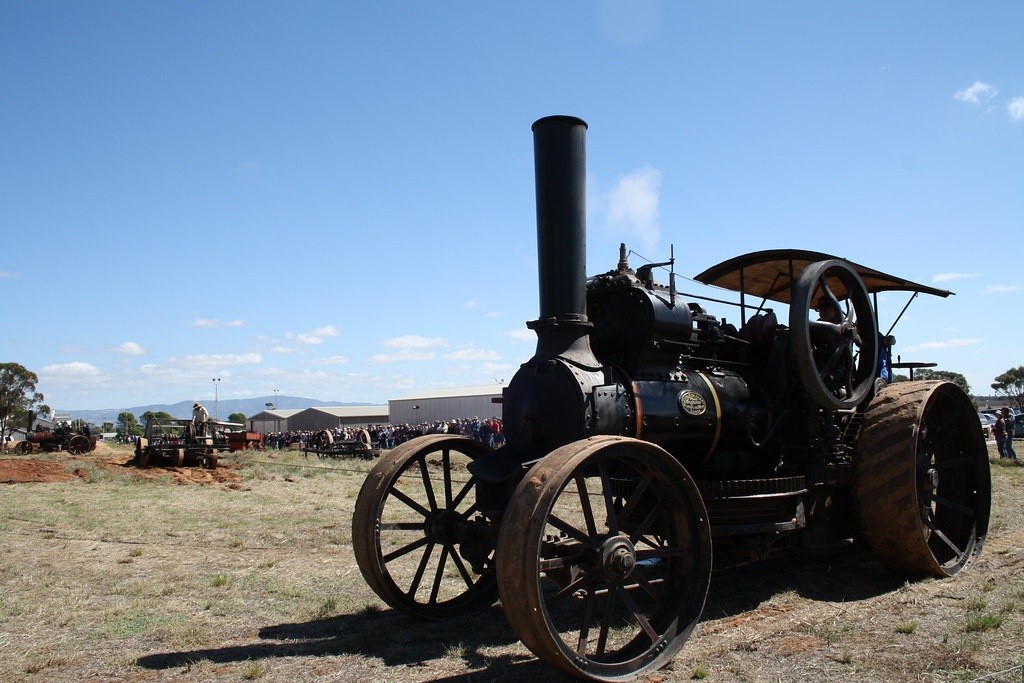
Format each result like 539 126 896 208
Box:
162 433 179 444
259 416 506 457
1001 408 1017 459
133 435 138 449
815 294 894 391
991 410 1008 459
62 420 69 429
83 424 92 451
191 402 208 444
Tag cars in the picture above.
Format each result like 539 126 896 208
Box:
977 407 1024 437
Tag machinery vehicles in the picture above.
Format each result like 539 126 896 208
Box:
135 412 230 469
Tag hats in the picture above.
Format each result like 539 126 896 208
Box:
193 402 201 408
451 419 457 423
473 416 478 419
995 409 1002 415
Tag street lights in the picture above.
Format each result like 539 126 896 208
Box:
212 378 220 421
274 389 279 402
